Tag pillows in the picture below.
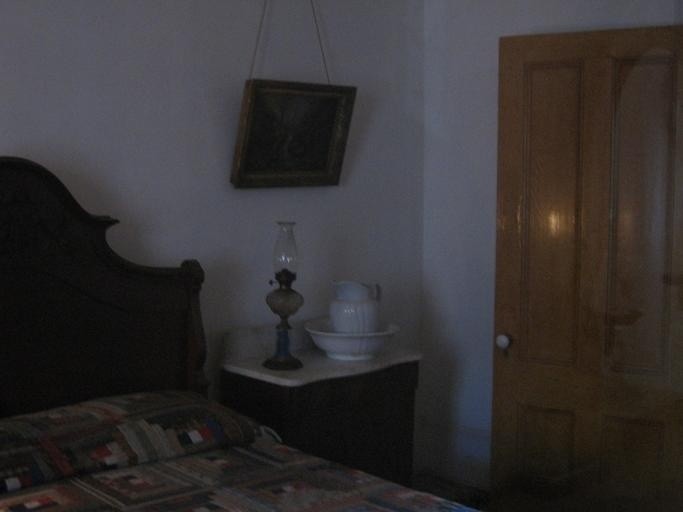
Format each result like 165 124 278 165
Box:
1 424 69 496
12 388 275 475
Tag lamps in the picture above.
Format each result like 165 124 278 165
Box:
264 218 304 369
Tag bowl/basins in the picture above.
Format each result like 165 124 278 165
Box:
303 314 400 362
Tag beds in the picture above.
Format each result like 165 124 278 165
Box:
1 155 477 512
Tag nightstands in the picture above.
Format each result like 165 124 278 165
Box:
221 342 420 488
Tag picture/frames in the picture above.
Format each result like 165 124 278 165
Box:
230 80 358 189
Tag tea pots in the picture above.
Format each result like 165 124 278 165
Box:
326 278 384 334
